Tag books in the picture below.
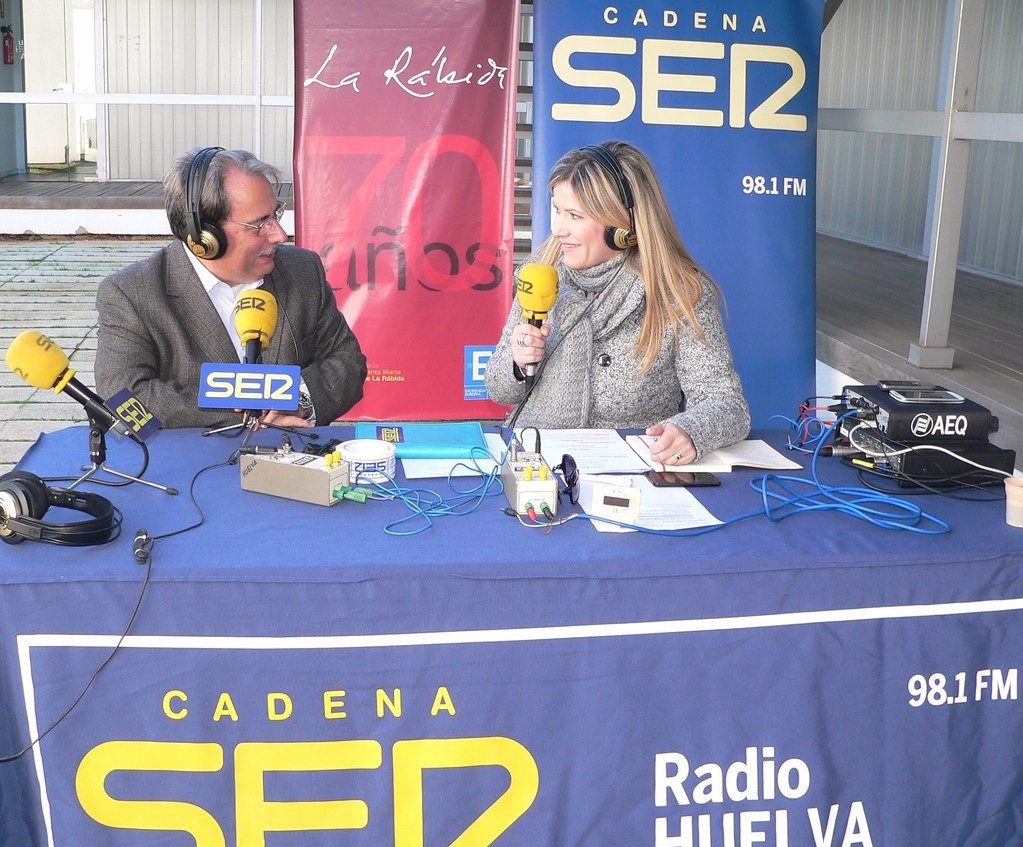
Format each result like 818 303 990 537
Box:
355 421 489 459
626 434 804 473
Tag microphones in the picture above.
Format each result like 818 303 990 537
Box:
5 330 143 443
517 263 559 398
234 290 278 415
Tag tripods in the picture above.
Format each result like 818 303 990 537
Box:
67 401 179 495
200 410 319 465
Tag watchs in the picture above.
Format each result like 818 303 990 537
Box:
297 392 312 422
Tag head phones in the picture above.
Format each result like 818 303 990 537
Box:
179 147 228 260
580 145 637 252
0 470 124 547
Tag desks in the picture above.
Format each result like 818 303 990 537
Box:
0 430 1023 847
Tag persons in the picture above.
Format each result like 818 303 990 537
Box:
485 138 752 467
94 146 368 430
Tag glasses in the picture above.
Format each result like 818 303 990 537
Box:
221 199 286 237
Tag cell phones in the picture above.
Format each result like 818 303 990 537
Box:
643 472 722 487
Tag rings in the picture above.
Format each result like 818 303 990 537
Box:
517 334 527 347
673 452 682 461
